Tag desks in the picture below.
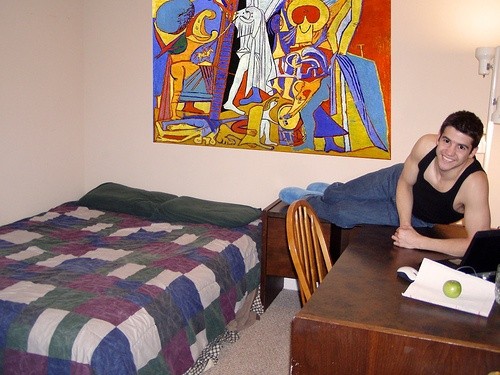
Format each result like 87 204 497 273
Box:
289 222 499 374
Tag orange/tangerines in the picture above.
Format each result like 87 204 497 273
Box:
444 279 463 299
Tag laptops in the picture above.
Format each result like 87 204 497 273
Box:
418 229 500 273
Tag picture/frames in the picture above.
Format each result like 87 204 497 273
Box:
152 0 391 161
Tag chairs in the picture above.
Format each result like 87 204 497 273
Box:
286 199 333 307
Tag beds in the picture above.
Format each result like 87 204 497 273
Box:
0 201 263 375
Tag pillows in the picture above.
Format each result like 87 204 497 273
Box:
148 195 262 228
77 182 178 218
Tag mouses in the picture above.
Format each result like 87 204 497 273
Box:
397 266 418 282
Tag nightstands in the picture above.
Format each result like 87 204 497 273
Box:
257 197 341 314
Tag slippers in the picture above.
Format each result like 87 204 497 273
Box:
307 182 330 193
279 186 324 204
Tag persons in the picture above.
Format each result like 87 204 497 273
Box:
278 110 491 258
